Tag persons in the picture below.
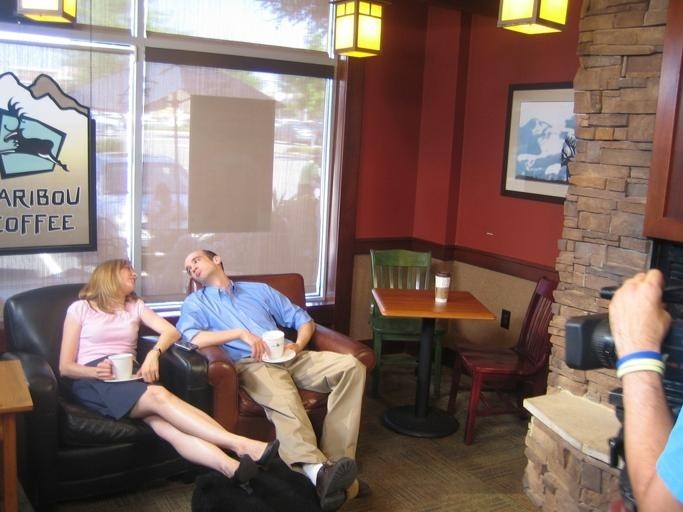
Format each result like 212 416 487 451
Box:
58 259 280 496
608 269 683 512
298 152 322 199
176 249 367 512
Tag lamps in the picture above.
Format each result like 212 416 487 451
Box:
15 1 77 24
497 0 569 33
330 0 392 59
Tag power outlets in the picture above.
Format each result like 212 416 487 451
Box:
501 309 510 330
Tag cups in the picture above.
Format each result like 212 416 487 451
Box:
262 330 285 360
434 271 451 303
108 353 133 380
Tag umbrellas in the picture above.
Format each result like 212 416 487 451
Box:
66 60 285 230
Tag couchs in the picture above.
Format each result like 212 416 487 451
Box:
190 272 377 463
1 285 210 511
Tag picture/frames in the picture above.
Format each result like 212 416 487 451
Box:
500 82 574 202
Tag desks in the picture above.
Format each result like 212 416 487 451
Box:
372 286 496 438
1 359 35 511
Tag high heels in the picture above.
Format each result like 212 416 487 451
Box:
254 439 280 472
230 453 258 498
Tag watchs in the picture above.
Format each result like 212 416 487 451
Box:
152 346 162 354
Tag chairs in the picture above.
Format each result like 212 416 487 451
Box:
448 277 565 445
370 250 447 400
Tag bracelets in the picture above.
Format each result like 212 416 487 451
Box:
614 351 670 381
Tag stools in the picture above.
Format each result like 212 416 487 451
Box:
191 466 323 511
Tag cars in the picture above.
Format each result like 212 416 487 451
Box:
92 153 202 262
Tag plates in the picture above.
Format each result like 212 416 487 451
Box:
103 374 143 383
260 348 296 364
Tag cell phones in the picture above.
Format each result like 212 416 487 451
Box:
175 342 199 353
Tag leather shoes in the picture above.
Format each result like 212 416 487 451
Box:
314 456 358 511
353 477 372 497
